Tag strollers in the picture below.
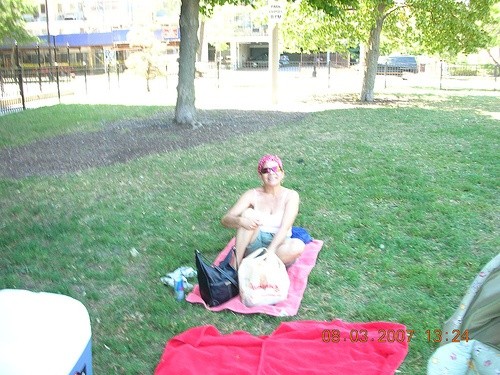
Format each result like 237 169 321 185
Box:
426 254 500 375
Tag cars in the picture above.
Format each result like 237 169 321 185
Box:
246 52 290 69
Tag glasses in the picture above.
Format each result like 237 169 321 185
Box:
261 166 279 173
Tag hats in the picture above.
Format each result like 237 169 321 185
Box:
258 153 282 173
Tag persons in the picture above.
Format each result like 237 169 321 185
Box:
222 154 306 270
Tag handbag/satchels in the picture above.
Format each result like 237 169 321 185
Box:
195 247 239 307
238 248 289 306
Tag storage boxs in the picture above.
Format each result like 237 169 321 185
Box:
0 289 94 375
